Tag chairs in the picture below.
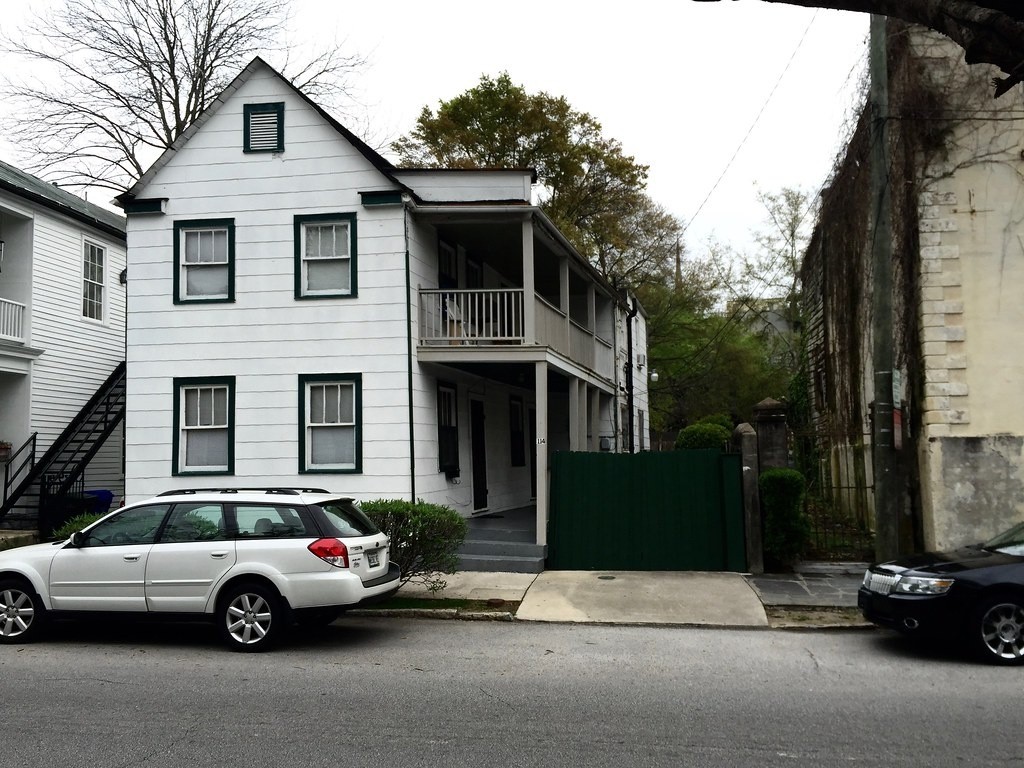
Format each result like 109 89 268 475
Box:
445 297 477 346
154 516 306 541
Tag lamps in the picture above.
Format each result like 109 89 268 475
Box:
647 369 658 381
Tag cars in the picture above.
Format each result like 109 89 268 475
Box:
857 521 1024 665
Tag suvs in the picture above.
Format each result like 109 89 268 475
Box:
0 488 402 654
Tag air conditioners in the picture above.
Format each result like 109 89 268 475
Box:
637 353 645 366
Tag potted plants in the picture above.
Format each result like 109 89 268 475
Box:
0 441 12 462
